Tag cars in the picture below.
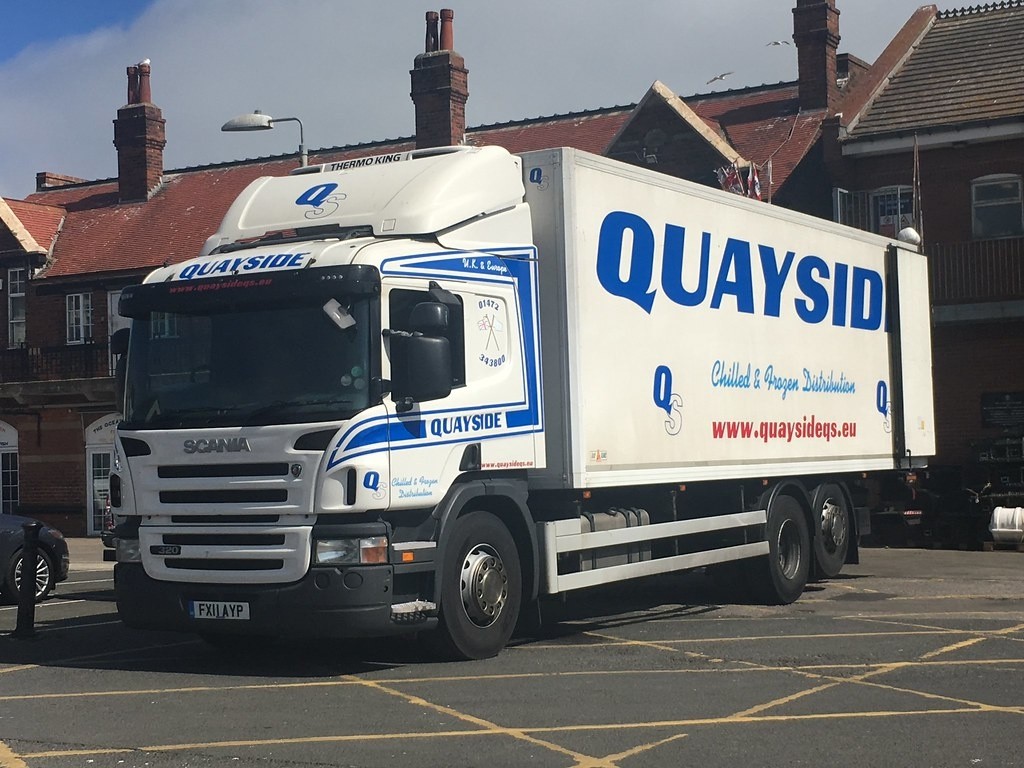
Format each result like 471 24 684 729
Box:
0 514 71 602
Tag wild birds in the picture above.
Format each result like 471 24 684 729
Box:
765 39 792 47
705 71 734 85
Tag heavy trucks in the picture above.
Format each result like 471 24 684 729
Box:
98 142 940 661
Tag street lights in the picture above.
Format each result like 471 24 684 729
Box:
219 108 310 169
